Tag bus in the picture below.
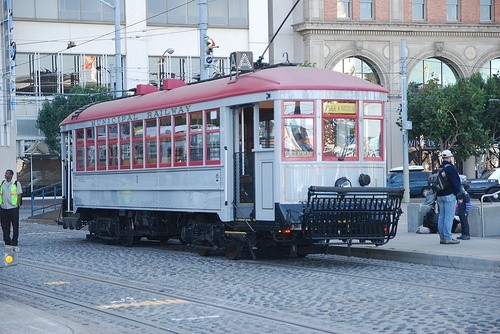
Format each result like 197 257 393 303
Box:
56 63 405 260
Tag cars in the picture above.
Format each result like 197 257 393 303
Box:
463 167 500 202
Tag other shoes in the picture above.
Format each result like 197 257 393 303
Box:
440 238 460 244
457 234 470 240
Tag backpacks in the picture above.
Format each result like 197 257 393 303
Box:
428 164 454 194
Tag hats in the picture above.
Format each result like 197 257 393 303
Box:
442 150 453 157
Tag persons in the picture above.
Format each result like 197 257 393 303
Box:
0 170 23 246
416 149 471 244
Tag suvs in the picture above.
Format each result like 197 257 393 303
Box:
384 166 432 198
